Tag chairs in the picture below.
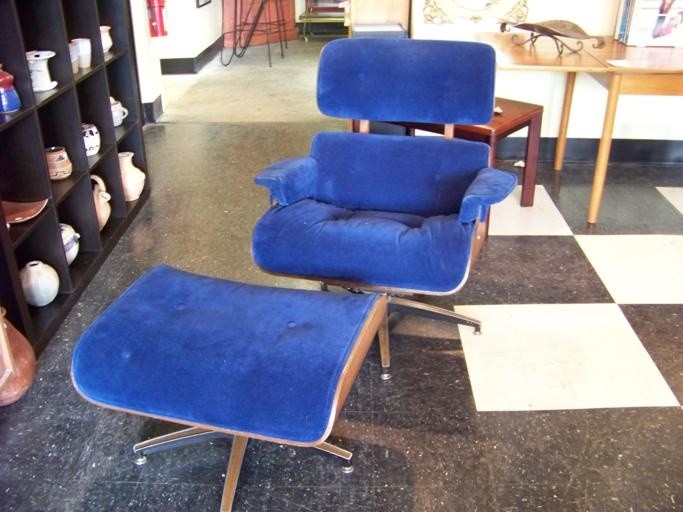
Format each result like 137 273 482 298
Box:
252 37 518 382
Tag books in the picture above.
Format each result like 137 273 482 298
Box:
609 0 682 50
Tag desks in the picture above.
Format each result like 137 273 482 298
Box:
470 31 683 224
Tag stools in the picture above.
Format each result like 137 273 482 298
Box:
71 260 389 511
387 95 544 208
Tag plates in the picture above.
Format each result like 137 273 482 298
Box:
500 20 606 56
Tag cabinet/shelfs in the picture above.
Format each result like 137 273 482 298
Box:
1 1 151 408
